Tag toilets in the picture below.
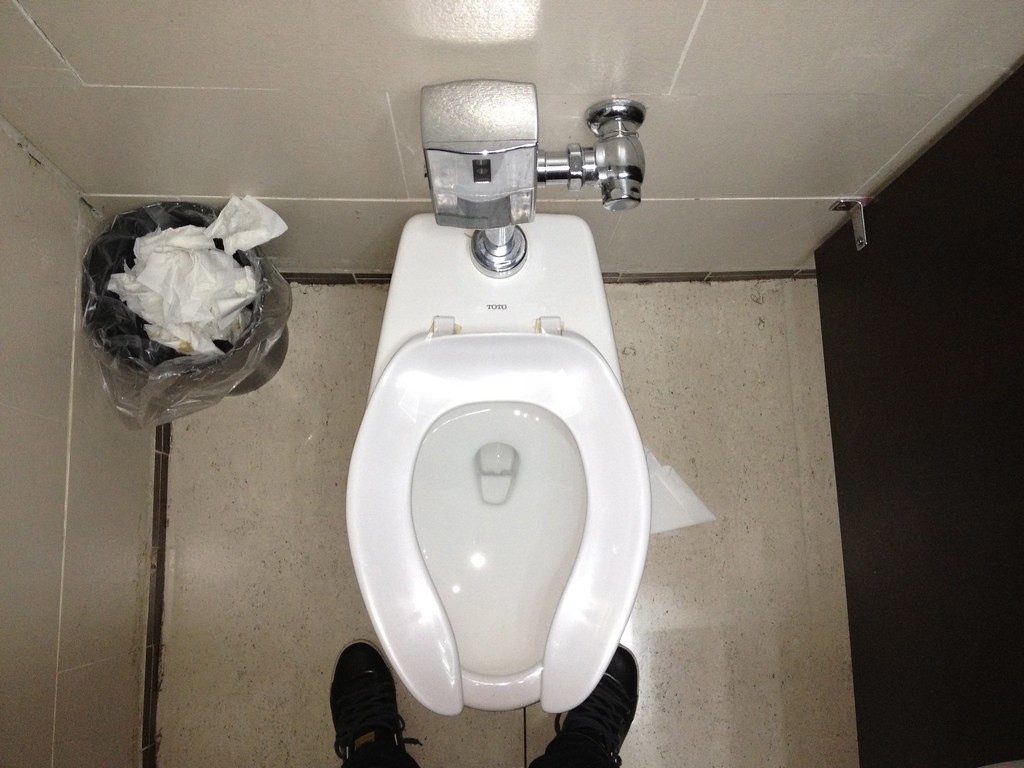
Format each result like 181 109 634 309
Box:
343 209 652 719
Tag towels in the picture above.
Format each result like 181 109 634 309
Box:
420 79 539 142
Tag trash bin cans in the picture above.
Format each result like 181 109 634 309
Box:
80 203 290 396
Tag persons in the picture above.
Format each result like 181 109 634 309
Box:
328 637 639 768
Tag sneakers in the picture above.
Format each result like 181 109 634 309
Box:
555 643 640 768
329 639 422 762
